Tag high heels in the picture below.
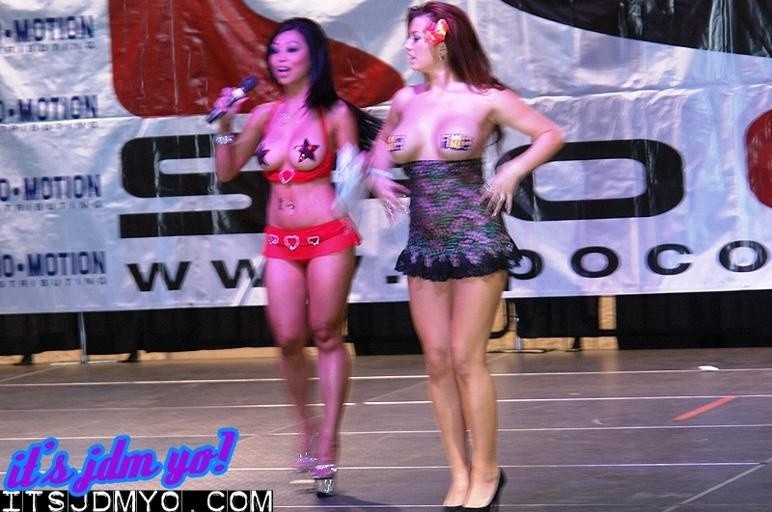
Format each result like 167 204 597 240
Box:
296 427 337 497
442 467 507 511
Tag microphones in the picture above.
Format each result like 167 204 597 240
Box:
206 75 259 125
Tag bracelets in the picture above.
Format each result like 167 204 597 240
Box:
211 133 235 145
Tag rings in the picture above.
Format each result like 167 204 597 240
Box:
498 195 506 202
383 199 391 208
483 183 493 192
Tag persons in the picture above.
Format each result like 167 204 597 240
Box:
363 1 571 511
208 15 366 500
